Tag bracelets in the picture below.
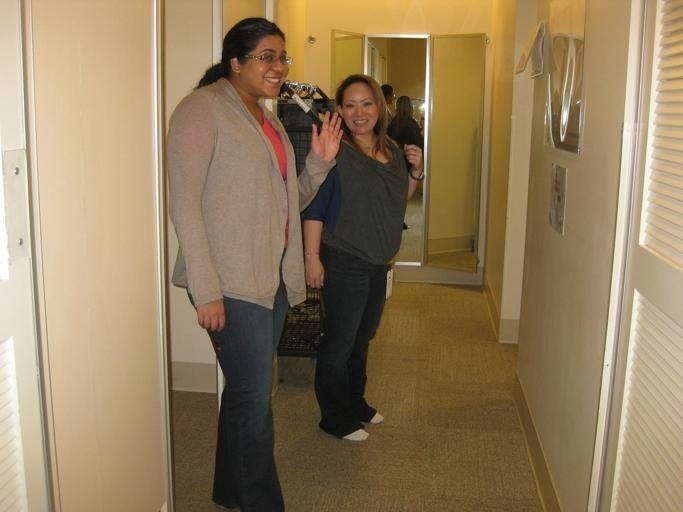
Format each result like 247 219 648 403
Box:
409 170 424 182
303 249 319 255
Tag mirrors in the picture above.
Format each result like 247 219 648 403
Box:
332 28 365 100
365 32 430 270
423 32 487 274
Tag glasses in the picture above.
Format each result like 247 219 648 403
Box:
246 53 293 65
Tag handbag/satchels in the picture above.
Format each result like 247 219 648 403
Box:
277 290 323 357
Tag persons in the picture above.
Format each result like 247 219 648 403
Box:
299 73 426 446
379 84 397 122
387 95 423 230
166 16 345 511
